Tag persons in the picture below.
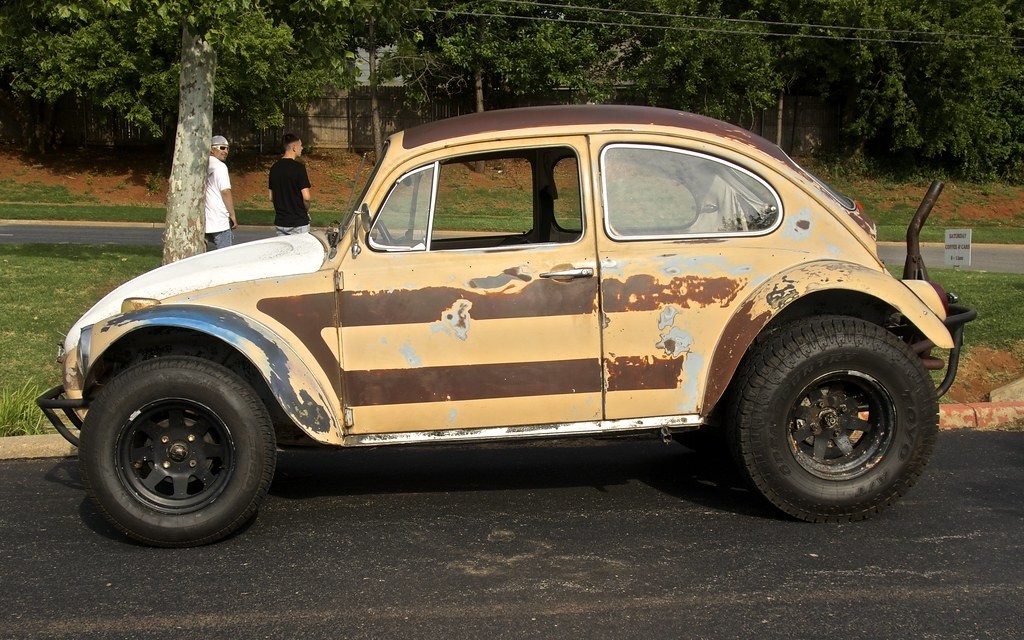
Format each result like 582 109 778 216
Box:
268 134 312 237
204 135 237 252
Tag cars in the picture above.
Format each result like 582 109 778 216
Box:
32 105 975 548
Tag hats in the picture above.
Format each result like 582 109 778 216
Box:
212 135 229 145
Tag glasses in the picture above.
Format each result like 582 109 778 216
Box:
220 146 231 150
